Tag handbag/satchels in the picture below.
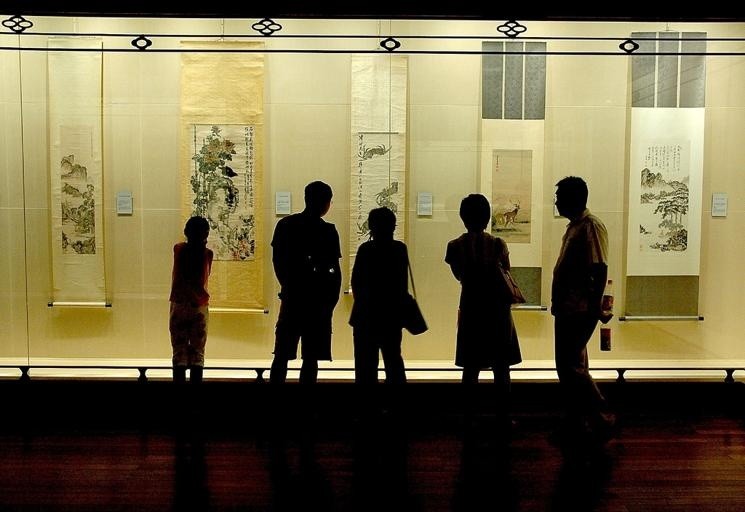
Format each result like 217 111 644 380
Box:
404 291 428 335
493 260 527 304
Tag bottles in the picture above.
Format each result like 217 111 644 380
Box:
600 322 612 352
600 280 615 317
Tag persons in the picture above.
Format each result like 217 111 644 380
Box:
267 181 342 384
167 214 215 387
348 206 410 384
444 193 523 446
549 176 624 444
